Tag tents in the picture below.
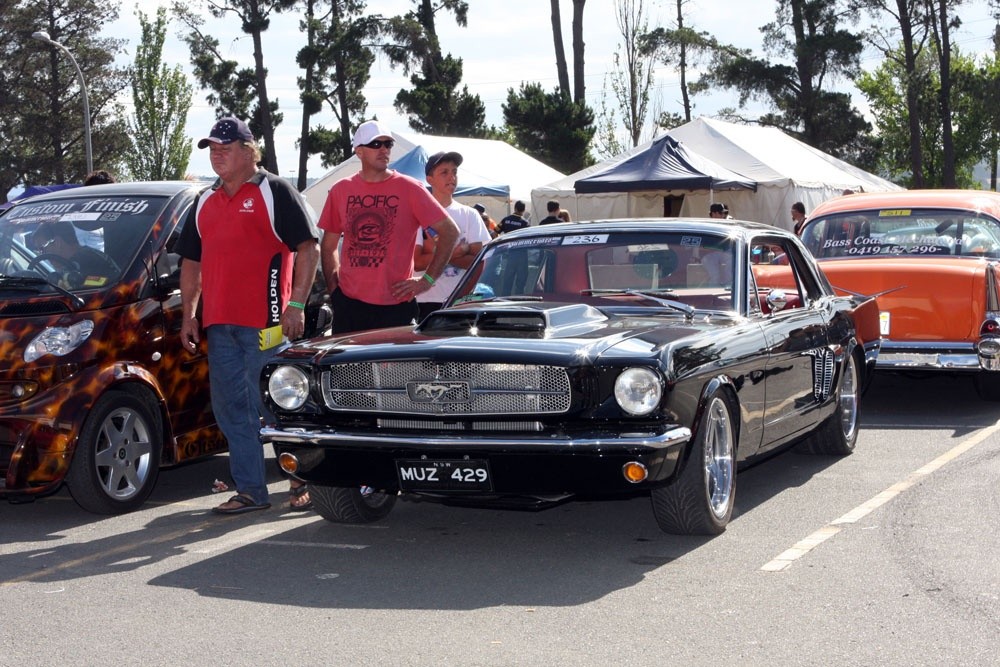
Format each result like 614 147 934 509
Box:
299 117 907 232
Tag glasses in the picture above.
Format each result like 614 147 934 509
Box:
358 140 394 149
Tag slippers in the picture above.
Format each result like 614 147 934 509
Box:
212 495 271 513
290 483 310 511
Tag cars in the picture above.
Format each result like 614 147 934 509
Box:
750 188 998 403
251 217 905 534
0 184 276 516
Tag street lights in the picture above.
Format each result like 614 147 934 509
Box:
31 30 92 176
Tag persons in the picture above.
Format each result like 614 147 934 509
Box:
478 213 496 239
492 199 531 298
536 199 565 261
790 201 809 238
71 169 116 251
472 203 499 232
693 202 735 287
172 117 330 517
315 120 461 337
31 219 123 288
413 151 492 324
558 209 570 226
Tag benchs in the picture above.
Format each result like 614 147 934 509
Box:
573 291 804 317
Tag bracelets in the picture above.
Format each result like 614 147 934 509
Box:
423 274 436 285
287 301 305 310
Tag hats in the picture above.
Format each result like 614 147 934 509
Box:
353 120 395 149
424 152 464 175
709 204 730 213
473 204 484 213
197 117 253 149
